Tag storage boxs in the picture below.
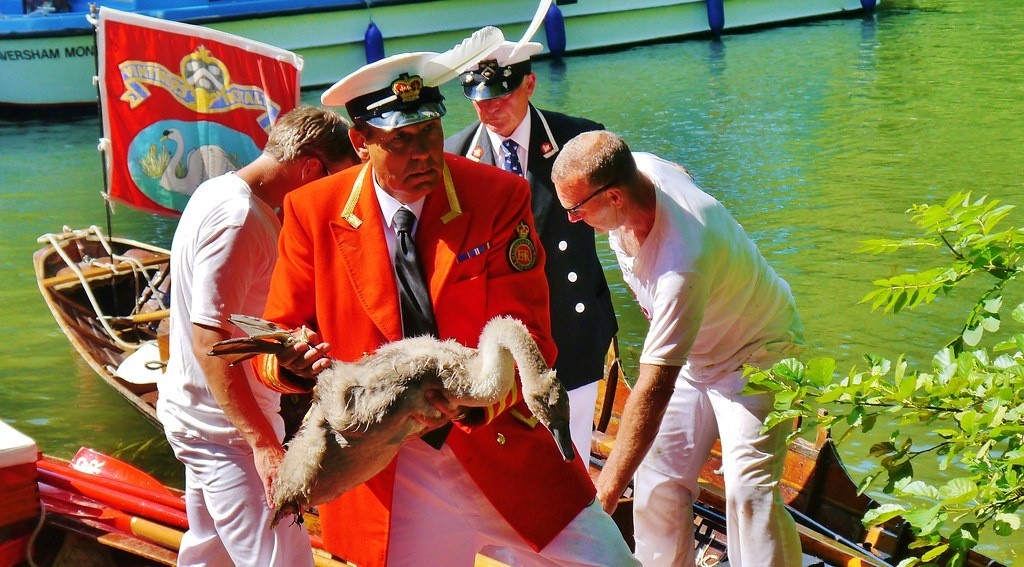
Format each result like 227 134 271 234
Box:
0 419 43 567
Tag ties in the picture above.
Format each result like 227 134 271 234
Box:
499 139 524 179
389 207 456 450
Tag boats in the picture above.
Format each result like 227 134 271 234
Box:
0 1 886 107
1 421 355 567
29 3 1008 567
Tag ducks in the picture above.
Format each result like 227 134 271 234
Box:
205 313 577 529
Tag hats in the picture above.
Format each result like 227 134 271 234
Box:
320 51 446 129
457 40 543 100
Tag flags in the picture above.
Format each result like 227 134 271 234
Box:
95 6 306 221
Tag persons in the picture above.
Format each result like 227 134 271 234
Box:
157 106 367 566
446 41 623 475
553 128 812 567
252 48 646 567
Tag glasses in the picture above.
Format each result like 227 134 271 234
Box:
565 179 619 218
